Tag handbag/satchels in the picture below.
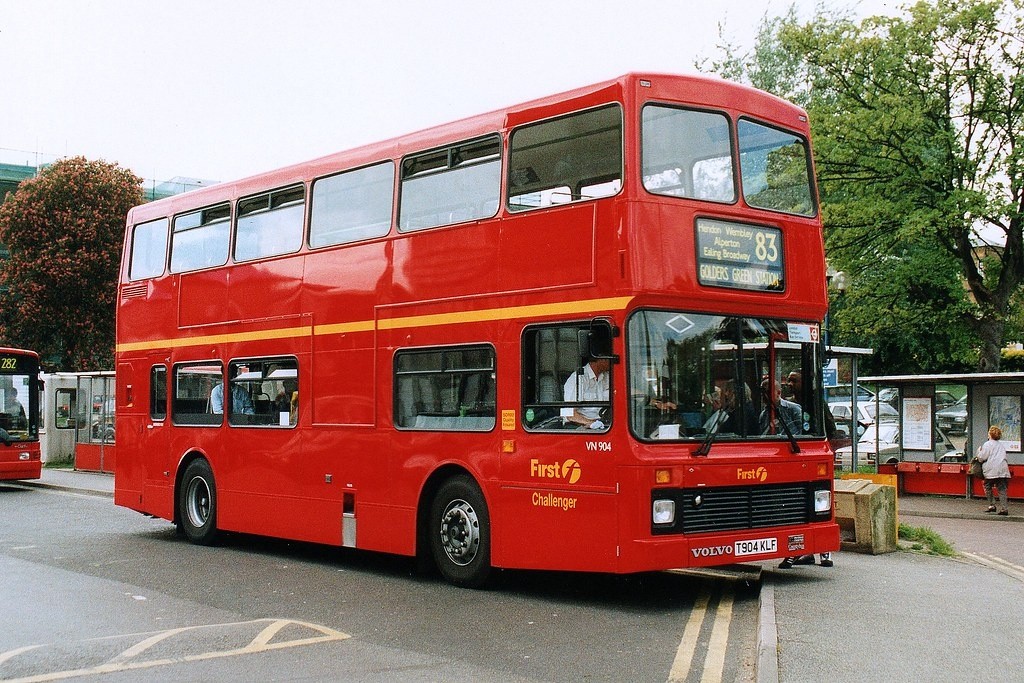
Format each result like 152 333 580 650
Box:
965 455 984 479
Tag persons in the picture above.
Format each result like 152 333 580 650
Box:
976 425 1012 515
556 331 678 430
5 388 27 418
780 368 837 566
209 362 256 414
758 378 802 570
709 378 761 436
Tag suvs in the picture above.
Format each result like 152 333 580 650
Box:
828 399 900 437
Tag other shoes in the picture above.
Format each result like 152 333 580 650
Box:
778 555 798 568
985 505 995 512
794 554 815 564
998 509 1008 515
820 551 832 566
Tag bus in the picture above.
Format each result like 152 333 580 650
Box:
0 347 45 481
114 73 842 591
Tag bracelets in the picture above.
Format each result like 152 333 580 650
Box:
653 401 662 407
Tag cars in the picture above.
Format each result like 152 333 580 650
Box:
934 390 959 414
824 383 875 404
868 387 899 411
833 423 955 471
934 395 971 435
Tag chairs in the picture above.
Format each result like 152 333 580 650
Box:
395 373 564 418
